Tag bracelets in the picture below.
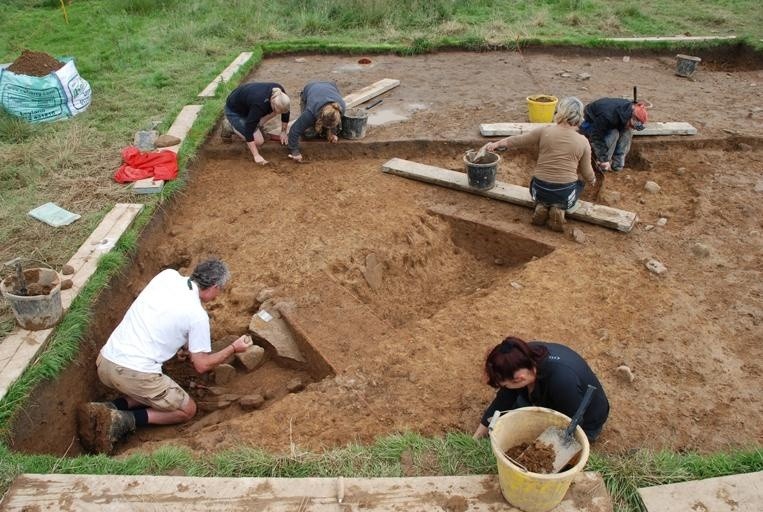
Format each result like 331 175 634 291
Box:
281 127 286 132
230 343 237 354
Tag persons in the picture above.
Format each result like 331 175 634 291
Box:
287 80 345 161
584 97 648 174
221 80 290 165
77 259 255 456
482 95 598 233
472 335 610 445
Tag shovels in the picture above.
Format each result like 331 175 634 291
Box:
515 384 597 474
288 154 312 164
471 142 506 162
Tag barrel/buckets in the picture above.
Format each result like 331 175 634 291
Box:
342 107 369 140
464 148 502 189
675 52 703 79
527 93 558 124
0 257 64 332
489 408 591 512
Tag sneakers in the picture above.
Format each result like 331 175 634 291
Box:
220 119 233 145
531 202 548 227
548 207 566 232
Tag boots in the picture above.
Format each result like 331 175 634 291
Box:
96 409 136 457
78 401 118 457
